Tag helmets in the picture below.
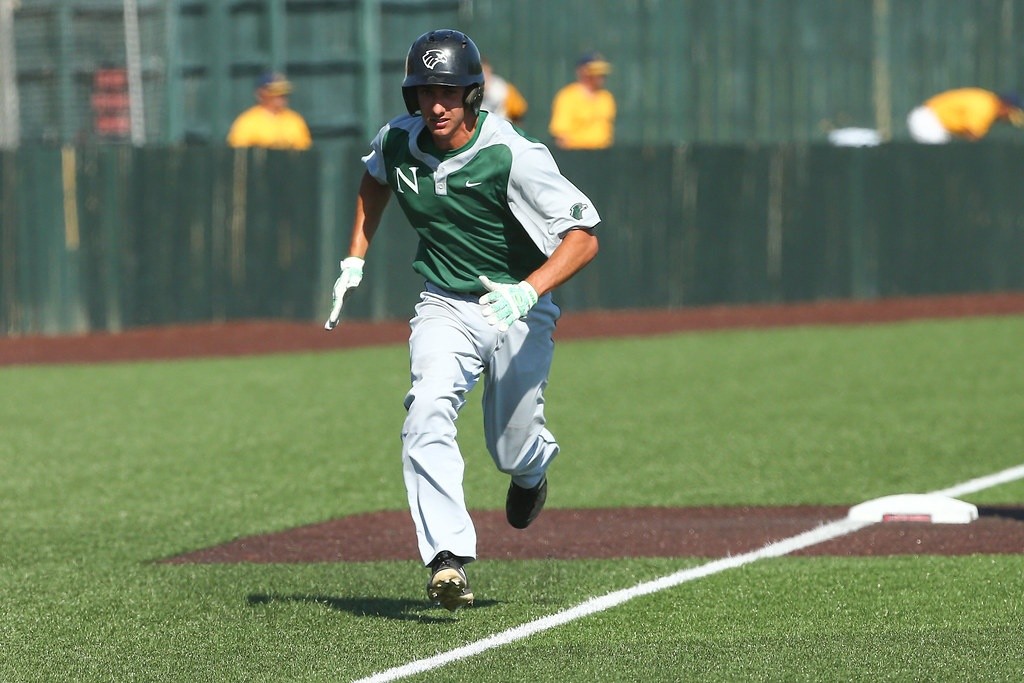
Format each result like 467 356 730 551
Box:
402 28 486 118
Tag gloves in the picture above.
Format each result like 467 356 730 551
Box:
477 275 540 331
325 256 368 332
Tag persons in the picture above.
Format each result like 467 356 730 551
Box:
225 69 315 152
323 26 603 614
480 57 530 123
547 51 619 150
906 84 1023 146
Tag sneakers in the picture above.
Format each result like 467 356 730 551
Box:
504 473 549 529
425 551 475 613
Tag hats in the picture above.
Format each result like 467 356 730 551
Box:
255 68 292 95
576 50 613 74
